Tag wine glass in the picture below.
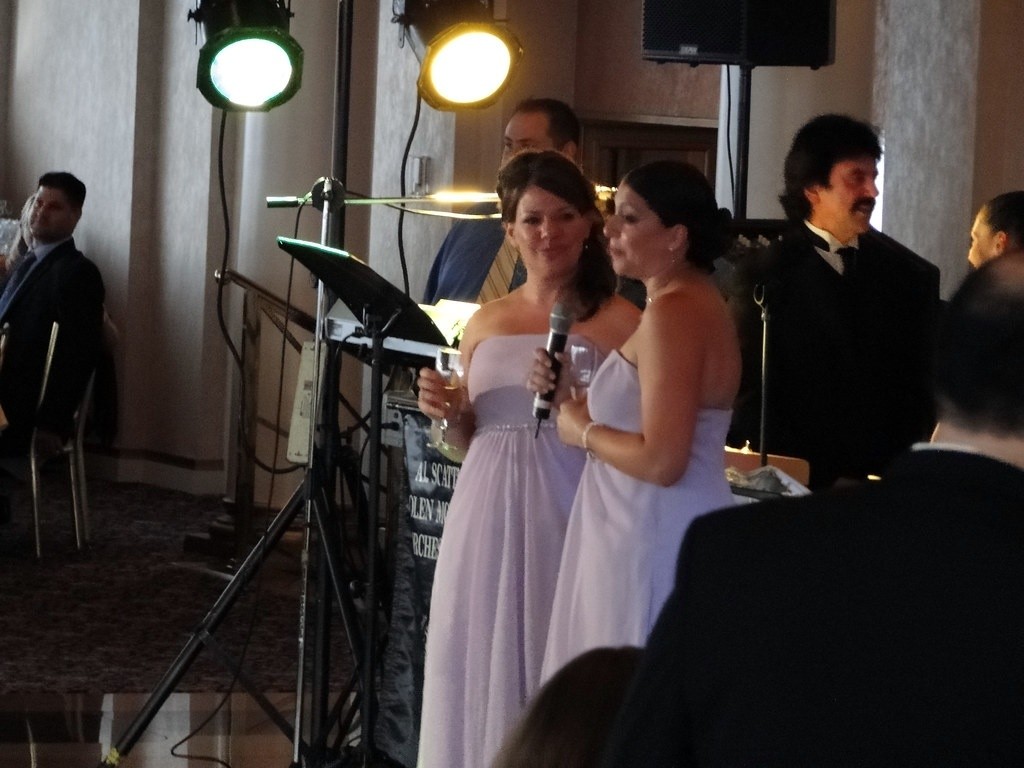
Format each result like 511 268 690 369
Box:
426 347 462 451
567 343 597 404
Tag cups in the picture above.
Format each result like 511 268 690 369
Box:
0 219 21 275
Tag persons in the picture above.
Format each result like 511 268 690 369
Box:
602 252 1024 768
0 173 106 524
967 188 1024 269
421 100 580 307
725 115 943 493
527 159 743 687
416 148 642 768
489 647 640 768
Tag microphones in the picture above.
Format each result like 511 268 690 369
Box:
742 259 781 323
533 298 576 439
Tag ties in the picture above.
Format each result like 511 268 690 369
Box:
0 249 37 318
800 220 867 292
475 231 519 305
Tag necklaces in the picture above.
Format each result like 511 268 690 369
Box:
646 293 662 302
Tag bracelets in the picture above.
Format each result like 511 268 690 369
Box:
582 421 601 449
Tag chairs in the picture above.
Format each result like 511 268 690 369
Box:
0 320 108 560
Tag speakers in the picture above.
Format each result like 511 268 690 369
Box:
640 0 838 71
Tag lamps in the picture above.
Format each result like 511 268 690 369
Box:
391 0 525 111
186 0 305 113
